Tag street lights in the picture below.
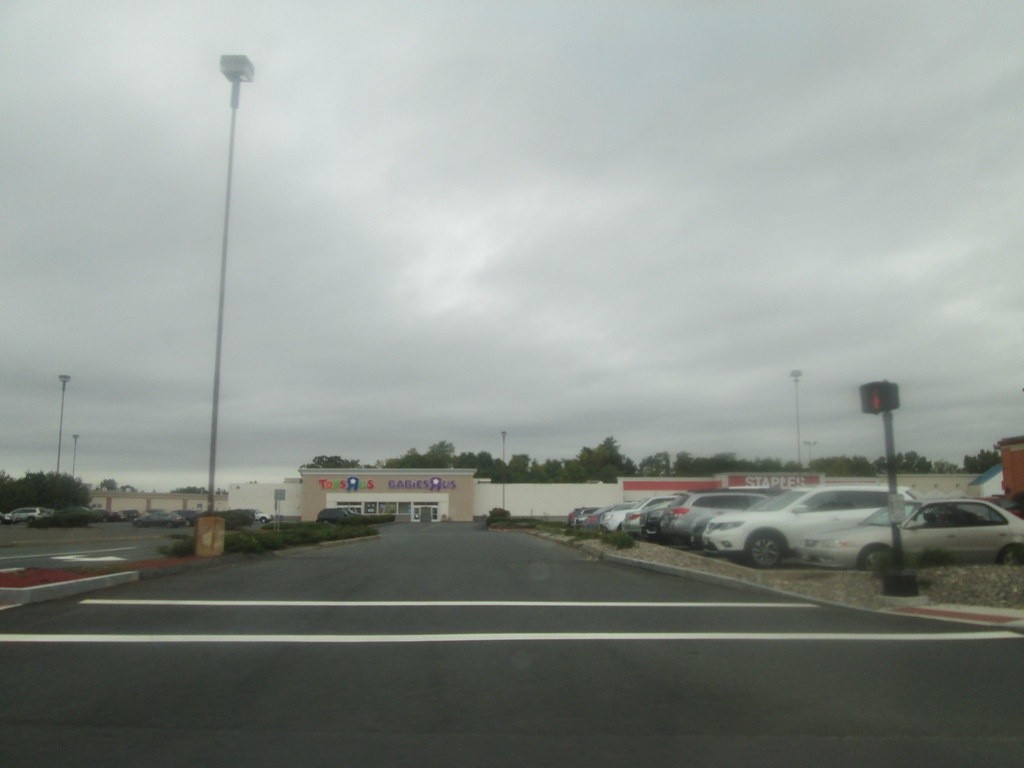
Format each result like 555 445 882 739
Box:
500 431 507 509
790 370 803 473
205 55 257 516
72 435 79 477
56 375 73 474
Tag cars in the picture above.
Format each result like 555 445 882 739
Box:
566 485 793 552
91 508 200 527
132 509 185 529
254 509 274 524
814 495 1024 572
0 506 55 525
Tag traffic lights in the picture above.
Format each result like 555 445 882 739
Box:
860 379 900 415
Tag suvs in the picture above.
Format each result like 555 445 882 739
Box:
701 482 929 571
316 507 359 526
